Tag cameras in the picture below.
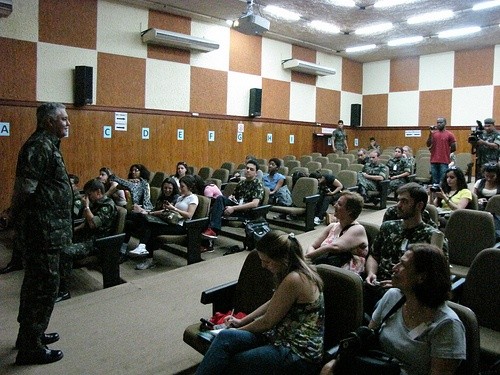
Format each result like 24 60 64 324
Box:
430 126 440 130
429 184 440 192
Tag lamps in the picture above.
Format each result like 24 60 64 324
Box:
283 58 337 77
141 28 220 54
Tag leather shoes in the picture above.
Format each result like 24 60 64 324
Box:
15 332 60 345
16 344 64 367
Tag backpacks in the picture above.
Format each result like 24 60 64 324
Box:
245 218 271 250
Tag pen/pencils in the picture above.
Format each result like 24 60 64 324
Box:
227 309 234 326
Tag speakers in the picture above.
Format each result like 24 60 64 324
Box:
250 88 261 116
75 65 93 106
351 104 361 127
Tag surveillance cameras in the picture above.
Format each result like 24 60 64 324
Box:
232 15 269 36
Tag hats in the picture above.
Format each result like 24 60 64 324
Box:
484 118 495 124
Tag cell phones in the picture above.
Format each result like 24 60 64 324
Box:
163 200 169 204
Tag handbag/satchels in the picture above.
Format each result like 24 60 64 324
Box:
159 210 179 225
274 185 293 206
335 326 399 375
312 252 352 267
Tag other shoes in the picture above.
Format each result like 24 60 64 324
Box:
129 248 149 257
120 245 128 255
0 262 25 274
137 260 154 270
201 228 218 240
200 245 216 254
286 215 294 221
313 217 322 225
362 194 369 202
55 294 71 303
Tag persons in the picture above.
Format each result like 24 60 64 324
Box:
12 102 76 363
358 152 386 200
374 147 411 205
427 167 473 231
473 161 500 211
321 244 467 375
194 173 222 199
234 155 310 225
332 120 348 153
305 192 369 273
56 159 199 302
309 169 344 224
368 137 381 154
426 118 456 184
469 118 500 182
363 183 449 319
202 161 264 251
197 231 325 375
403 146 415 169
358 149 371 165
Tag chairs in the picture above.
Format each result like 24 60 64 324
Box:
72 146 500 375
